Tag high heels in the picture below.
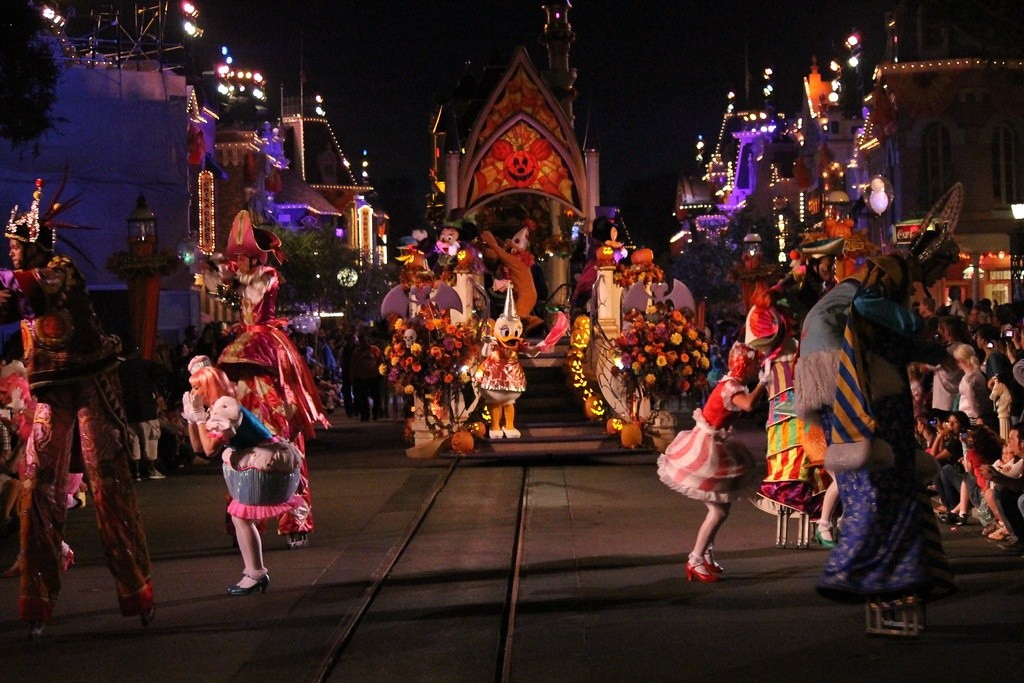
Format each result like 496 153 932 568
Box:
687 551 724 573
226 571 270 595
685 561 719 583
814 526 839 549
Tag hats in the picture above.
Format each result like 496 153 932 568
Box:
727 340 765 381
801 237 846 261
908 182 964 276
224 209 288 267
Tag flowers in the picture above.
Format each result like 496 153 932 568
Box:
372 309 480 410
602 304 711 397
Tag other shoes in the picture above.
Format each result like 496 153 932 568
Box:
3 554 21 577
29 619 46 641
997 536 1024 551
987 525 1009 540
932 504 947 513
938 510 967 527
174 466 192 475
191 457 210 465
147 469 165 480
982 521 998 536
133 471 143 482
526 317 545 333
58 549 76 571
139 606 155 626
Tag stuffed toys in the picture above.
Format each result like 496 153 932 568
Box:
481 219 544 334
412 222 479 321
474 286 570 438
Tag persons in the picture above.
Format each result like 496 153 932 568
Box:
286 324 390 421
0 326 240 576
0 178 156 641
656 255 844 582
183 355 302 596
908 285 1024 558
793 231 959 601
205 211 332 548
